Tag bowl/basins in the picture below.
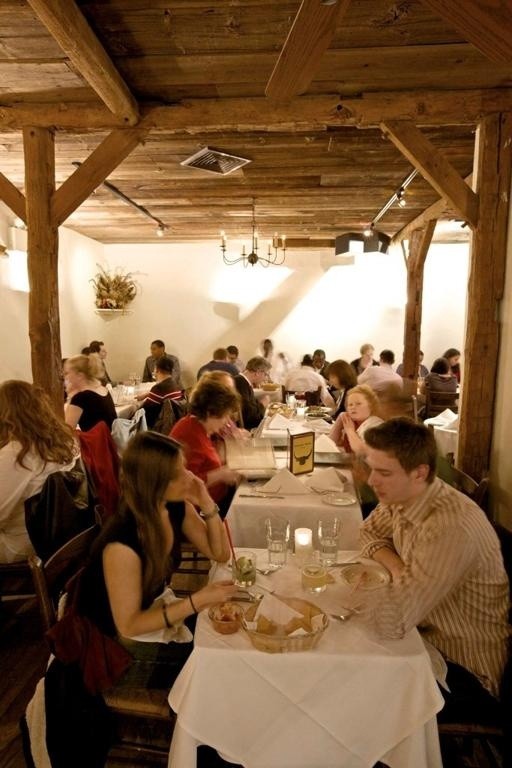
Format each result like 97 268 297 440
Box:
208 601 245 635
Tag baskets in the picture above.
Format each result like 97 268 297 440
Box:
243 595 329 653
262 383 279 390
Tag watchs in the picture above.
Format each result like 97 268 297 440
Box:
198 504 221 521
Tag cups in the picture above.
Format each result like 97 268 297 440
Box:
265 517 290 568
286 394 295 409
232 550 257 588
318 517 340 564
300 563 327 595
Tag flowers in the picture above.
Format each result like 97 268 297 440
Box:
87 262 148 316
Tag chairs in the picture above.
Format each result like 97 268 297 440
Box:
378 395 419 431
438 452 491 514
281 384 322 407
424 381 460 418
0 455 104 604
152 394 189 436
436 513 511 768
76 419 123 527
111 408 149 491
27 500 172 722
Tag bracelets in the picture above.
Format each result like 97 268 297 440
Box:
161 604 173 628
187 592 199 613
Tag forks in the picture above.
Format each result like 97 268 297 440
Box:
257 567 281 575
329 612 354 622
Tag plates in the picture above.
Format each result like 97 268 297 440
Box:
305 413 329 419
338 557 392 592
320 492 358 507
302 407 332 413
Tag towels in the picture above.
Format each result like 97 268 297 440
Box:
302 418 331 430
258 467 314 495
314 433 341 453
265 413 296 430
304 466 349 493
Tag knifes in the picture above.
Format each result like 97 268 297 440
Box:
239 494 285 499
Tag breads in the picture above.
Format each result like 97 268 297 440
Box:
286 618 310 633
257 615 275 635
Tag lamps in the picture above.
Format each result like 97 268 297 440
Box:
157 222 168 239
362 222 377 238
393 185 409 211
218 195 288 268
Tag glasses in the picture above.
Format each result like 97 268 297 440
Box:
258 368 270 379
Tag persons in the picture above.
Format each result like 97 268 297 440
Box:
372 382 453 490
77 431 240 688
64 353 117 434
226 345 239 370
91 341 114 387
397 350 429 378
286 354 336 407
311 349 331 375
357 416 511 721
357 350 403 394
143 340 182 384
1 380 89 592
419 358 458 420
188 370 251 441
231 356 272 433
168 381 248 543
198 348 241 379
350 344 380 376
257 338 293 403
328 385 387 520
442 349 461 384
322 360 359 423
134 357 186 431
82 347 97 355
